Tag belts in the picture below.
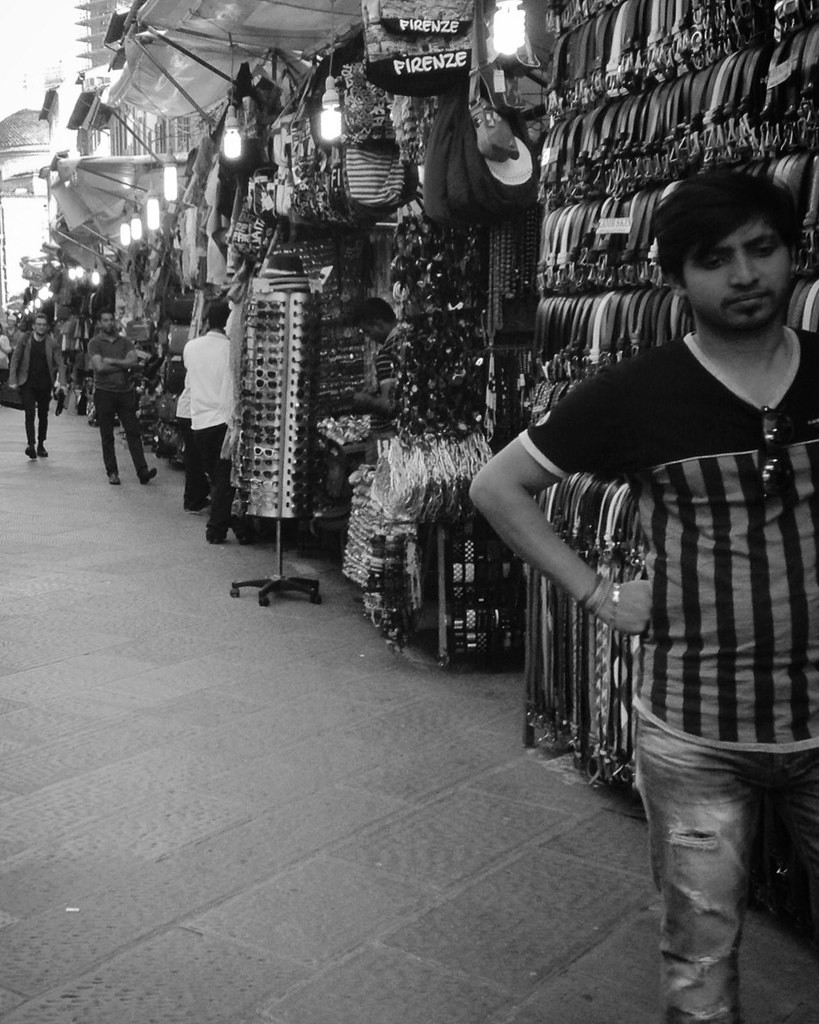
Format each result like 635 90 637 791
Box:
516 0 819 950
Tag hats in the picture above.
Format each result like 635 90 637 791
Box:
262 253 312 282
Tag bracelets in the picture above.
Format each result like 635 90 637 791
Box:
578 572 621 630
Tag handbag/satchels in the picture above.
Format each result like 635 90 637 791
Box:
182 0 550 235
18 233 193 471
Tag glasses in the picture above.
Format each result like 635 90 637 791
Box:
758 406 796 499
35 322 48 326
229 289 325 522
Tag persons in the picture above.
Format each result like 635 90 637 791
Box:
0 298 398 546
467 175 819 1023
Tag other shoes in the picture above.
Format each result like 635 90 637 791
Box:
240 534 258 545
25 446 37 460
187 497 214 511
139 466 157 485
108 473 121 485
37 445 49 457
208 536 225 545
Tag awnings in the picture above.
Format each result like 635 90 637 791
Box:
49 0 358 278
